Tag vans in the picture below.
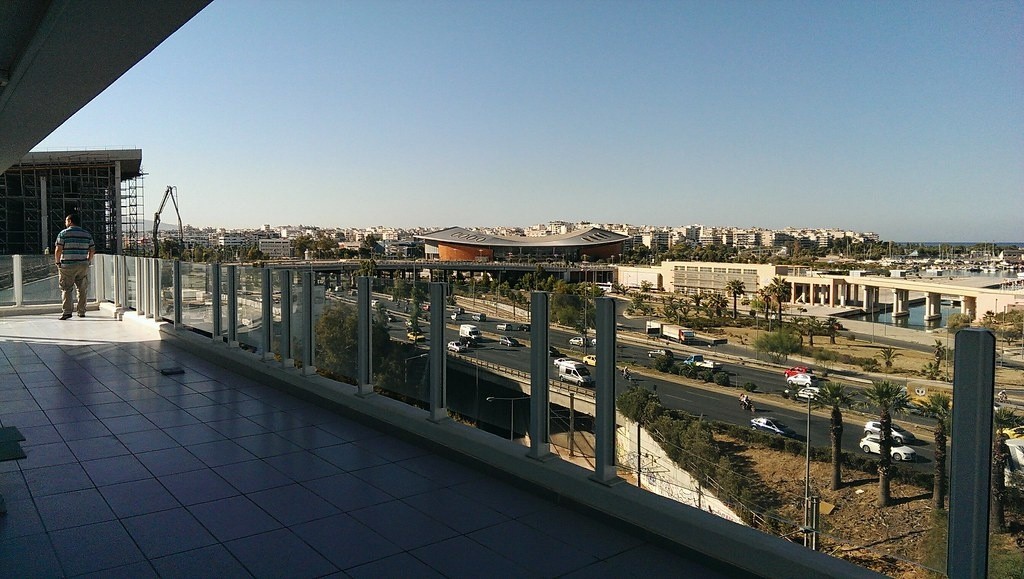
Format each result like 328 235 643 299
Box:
558 362 594 387
472 313 487 321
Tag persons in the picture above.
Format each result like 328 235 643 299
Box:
624 366 629 375
55 214 95 320
998 390 1006 400
740 394 751 409
45 247 50 254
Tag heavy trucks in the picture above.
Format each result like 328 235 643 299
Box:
662 324 695 344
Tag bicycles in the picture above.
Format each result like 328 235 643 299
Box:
995 396 1011 405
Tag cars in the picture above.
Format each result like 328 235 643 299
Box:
407 332 426 342
420 305 430 311
786 374 819 387
517 324 531 332
795 387 824 401
499 337 520 347
448 341 467 352
582 355 596 367
459 337 478 348
569 337 589 347
859 434 916 461
407 326 423 335
995 426 1024 439
496 323 512 331
446 305 465 320
648 349 674 359
553 358 571 369
750 416 797 438
863 420 916 445
784 367 812 378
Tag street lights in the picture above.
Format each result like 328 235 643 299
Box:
487 397 514 442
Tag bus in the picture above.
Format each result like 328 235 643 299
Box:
596 282 617 293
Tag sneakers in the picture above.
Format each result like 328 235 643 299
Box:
59 314 72 320
77 313 85 317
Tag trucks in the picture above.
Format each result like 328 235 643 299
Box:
684 355 723 373
894 380 955 419
458 324 483 342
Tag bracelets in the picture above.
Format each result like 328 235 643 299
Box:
56 262 60 265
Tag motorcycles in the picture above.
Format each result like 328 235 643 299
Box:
740 400 756 413
621 369 632 381
